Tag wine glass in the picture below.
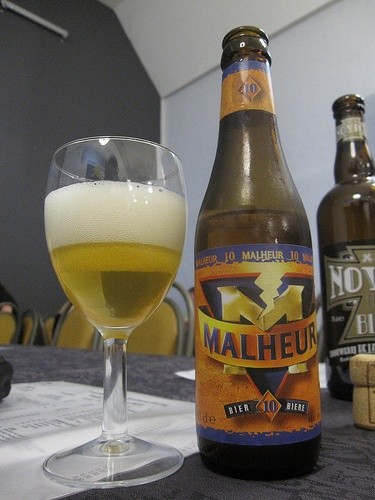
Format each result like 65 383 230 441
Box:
43 135 188 490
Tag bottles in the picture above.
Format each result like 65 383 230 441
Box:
193 26 324 481
315 95 375 402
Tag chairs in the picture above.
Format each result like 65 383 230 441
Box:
0 281 325 363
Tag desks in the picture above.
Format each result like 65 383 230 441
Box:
0 345 375 500
